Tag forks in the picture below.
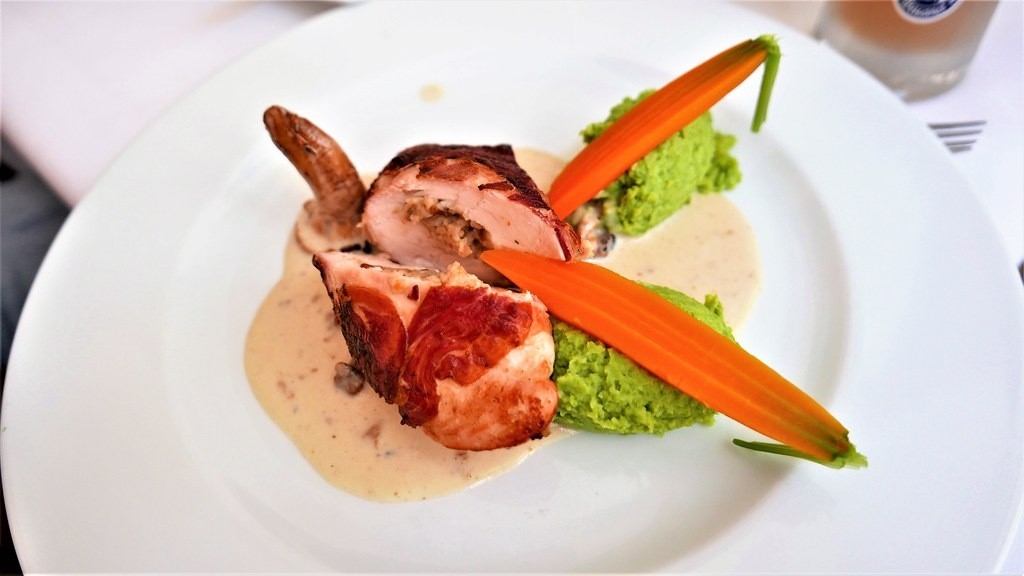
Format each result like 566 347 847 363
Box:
926 114 987 154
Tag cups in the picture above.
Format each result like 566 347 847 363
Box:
806 0 1003 104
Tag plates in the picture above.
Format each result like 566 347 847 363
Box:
0 0 1023 574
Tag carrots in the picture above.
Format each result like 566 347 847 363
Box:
481 249 866 471
546 35 781 220
